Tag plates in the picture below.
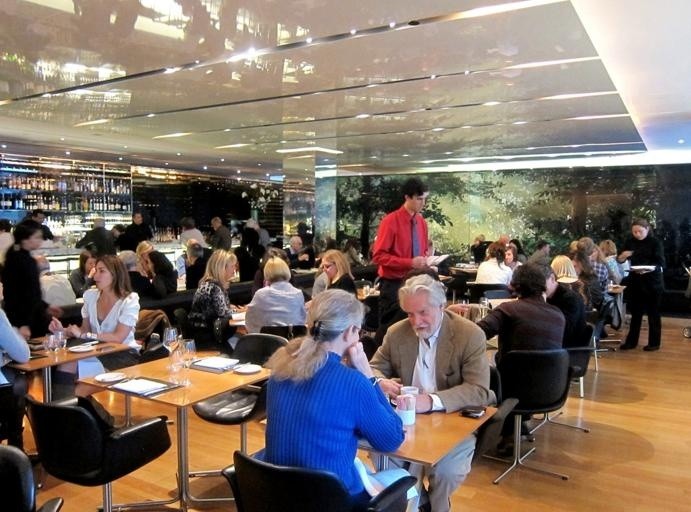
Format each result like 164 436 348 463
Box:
233 365 262 374
94 372 127 383
630 265 657 270
66 345 94 353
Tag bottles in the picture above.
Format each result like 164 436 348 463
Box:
1 173 132 212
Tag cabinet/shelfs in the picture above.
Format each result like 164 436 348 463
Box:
1 156 133 214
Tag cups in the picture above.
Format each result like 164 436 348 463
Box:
41 328 70 355
455 298 471 307
478 296 490 319
396 383 420 427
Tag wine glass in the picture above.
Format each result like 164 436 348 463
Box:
162 326 196 388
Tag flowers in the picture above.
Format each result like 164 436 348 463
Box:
241 181 280 211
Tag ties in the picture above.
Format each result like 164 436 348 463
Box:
410 218 421 257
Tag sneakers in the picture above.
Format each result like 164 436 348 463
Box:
618 341 638 350
643 343 661 351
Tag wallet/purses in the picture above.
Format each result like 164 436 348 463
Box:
461 404 487 418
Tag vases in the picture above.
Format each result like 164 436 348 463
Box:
250 210 259 222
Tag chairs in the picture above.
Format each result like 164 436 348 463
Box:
2 442 64 511
482 347 570 485
1 383 21 441
176 333 289 502
361 295 381 333
222 451 420 511
587 308 621 371
24 393 180 512
466 281 509 302
175 308 213 349
530 346 592 435
472 367 520 463
258 324 308 338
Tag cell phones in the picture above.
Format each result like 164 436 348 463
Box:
461 406 486 418
